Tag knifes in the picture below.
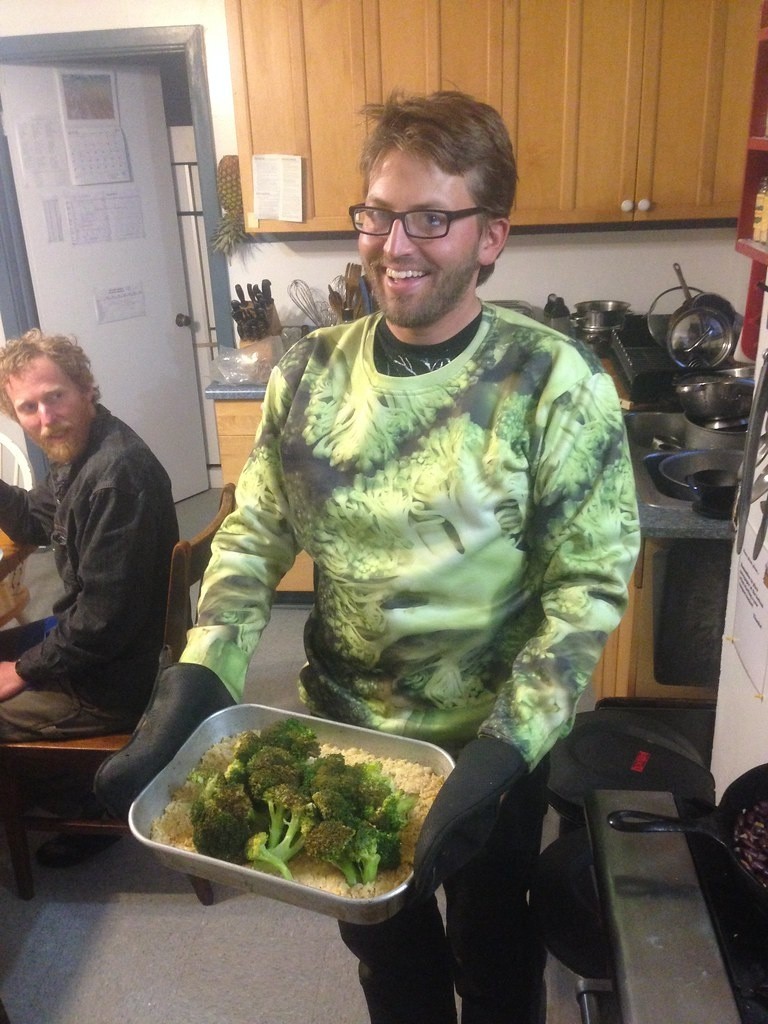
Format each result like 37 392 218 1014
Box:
231 280 281 341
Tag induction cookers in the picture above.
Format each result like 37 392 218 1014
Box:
606 328 675 402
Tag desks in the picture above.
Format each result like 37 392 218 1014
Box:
0 528 37 630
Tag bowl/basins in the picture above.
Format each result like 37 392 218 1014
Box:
659 452 743 488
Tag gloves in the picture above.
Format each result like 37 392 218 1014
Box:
413 737 526 901
96 664 237 827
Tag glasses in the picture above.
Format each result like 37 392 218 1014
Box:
349 203 483 239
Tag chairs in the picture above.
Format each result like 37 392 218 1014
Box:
1 481 236 907
1 433 35 494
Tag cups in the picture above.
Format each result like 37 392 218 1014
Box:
685 469 738 508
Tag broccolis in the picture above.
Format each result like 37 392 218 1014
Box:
191 720 418 885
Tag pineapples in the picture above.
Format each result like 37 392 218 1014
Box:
207 155 256 260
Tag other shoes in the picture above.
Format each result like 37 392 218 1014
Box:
38 819 124 865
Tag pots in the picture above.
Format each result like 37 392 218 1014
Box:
671 263 736 339
607 763 768 889
683 410 748 451
570 300 630 357
675 379 755 430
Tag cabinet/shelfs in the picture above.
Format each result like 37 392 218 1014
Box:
223 0 761 237
593 537 733 707
737 0 768 357
214 401 315 592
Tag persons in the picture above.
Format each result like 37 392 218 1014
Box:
90 88 644 1023
0 328 192 867
